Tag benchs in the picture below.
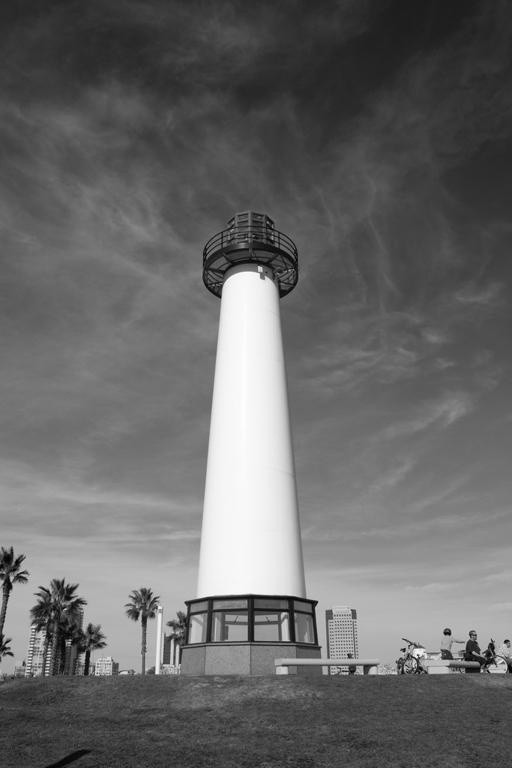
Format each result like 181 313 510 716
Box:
274 657 381 676
424 660 481 676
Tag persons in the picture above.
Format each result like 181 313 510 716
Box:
483 637 496 667
496 639 512 672
346 653 357 675
441 627 464 660
466 628 487 675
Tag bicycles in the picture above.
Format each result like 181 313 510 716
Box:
459 637 508 675
396 638 424 675
